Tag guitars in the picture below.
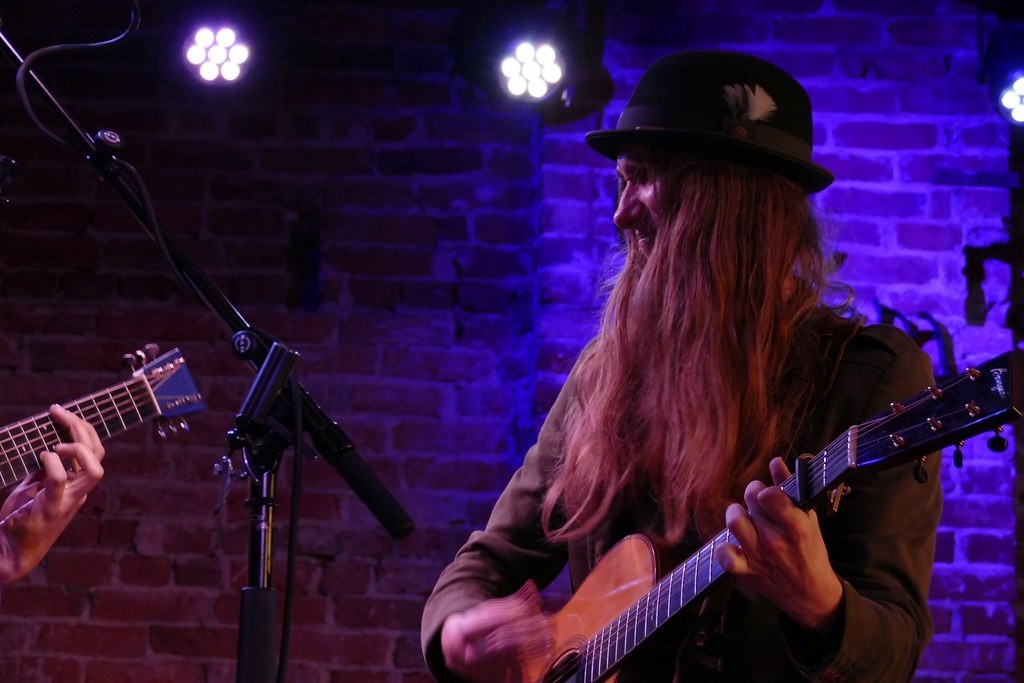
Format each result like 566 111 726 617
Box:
1 339 207 492
505 348 1024 683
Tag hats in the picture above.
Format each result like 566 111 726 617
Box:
585 50 835 194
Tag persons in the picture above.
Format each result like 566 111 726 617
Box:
0 403 107 590
420 45 938 682
